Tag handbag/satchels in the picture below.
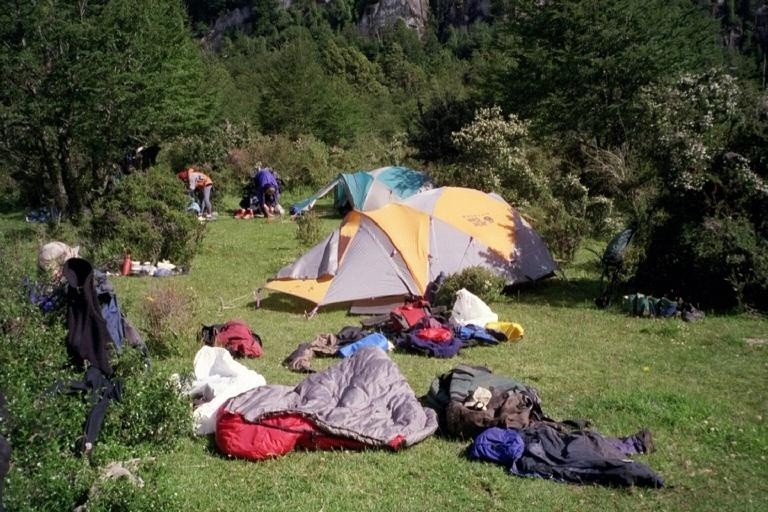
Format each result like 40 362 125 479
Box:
621 292 677 317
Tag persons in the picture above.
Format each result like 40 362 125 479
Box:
24 241 121 458
179 168 213 215
255 170 280 218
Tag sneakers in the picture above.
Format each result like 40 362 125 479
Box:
233 208 254 220
681 303 706 322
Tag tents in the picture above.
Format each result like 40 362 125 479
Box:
290 166 436 217
255 186 557 318
601 197 765 312
29 265 150 396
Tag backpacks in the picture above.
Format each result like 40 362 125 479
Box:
425 363 541 410
